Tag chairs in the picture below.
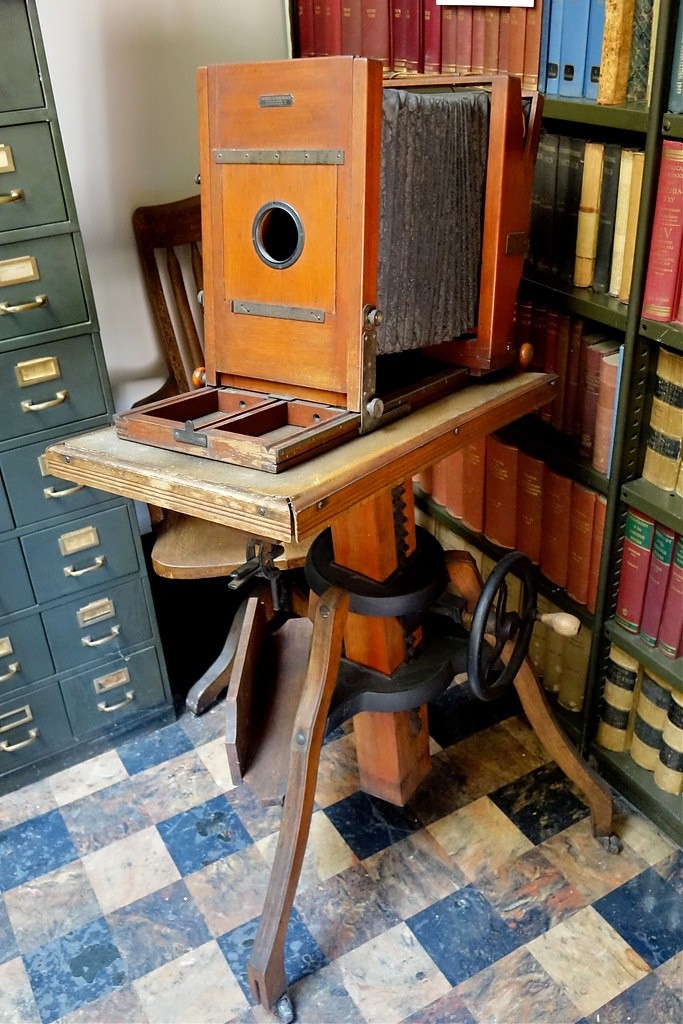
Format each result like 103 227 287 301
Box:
129 194 338 715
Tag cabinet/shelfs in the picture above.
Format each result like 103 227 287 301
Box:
286 1 682 847
1 0 179 783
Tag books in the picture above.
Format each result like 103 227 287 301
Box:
413 508 592 714
613 508 683 661
526 133 683 323
597 644 683 796
412 436 607 613
297 0 682 114
516 302 683 498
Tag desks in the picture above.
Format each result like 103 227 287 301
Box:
40 372 617 1024
